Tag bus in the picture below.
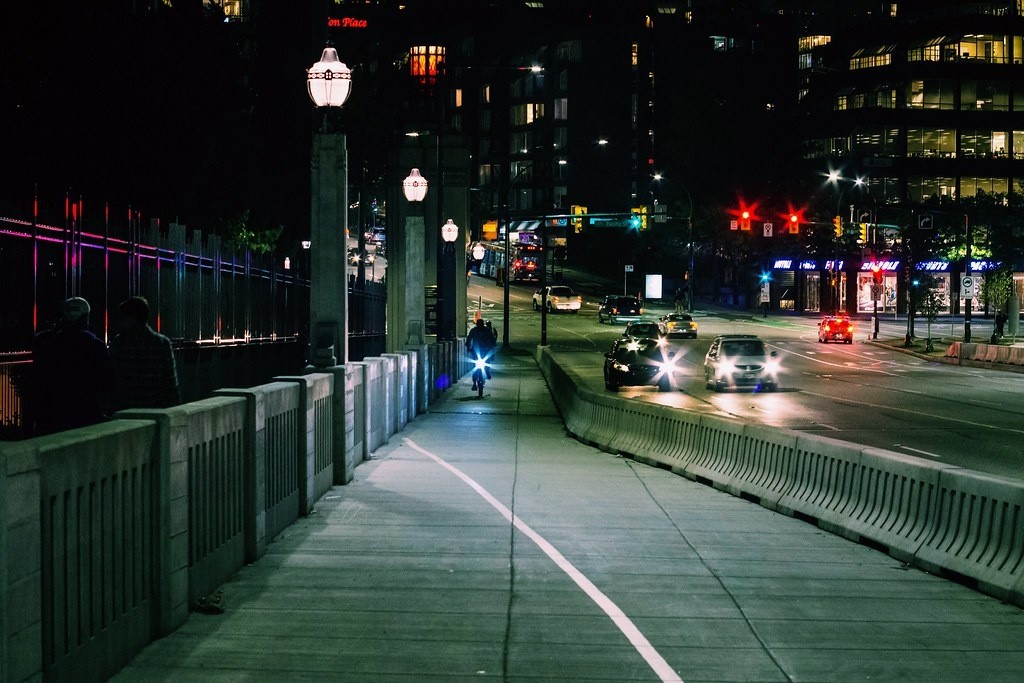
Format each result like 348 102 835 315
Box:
478 240 542 285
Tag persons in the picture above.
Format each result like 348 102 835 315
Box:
29 296 107 438
107 297 179 414
486 321 498 343
465 319 496 379
674 287 685 314
996 311 1008 338
990 330 999 344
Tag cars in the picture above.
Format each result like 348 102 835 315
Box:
598 295 643 325
364 227 386 256
621 319 662 343
603 338 675 392
817 315 853 345
703 334 778 392
346 248 372 266
659 313 698 340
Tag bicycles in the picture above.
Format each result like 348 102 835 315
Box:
468 348 493 398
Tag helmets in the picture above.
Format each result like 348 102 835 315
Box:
120 296 151 316
58 296 91 328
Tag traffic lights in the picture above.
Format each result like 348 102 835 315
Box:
832 215 841 238
857 209 873 225
859 223 869 244
741 210 751 230
788 212 799 235
918 215 933 230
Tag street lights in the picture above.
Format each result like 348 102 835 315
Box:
303 46 354 367
404 165 429 349
654 173 693 313
442 218 459 341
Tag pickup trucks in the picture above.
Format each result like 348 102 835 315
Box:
533 285 581 313
